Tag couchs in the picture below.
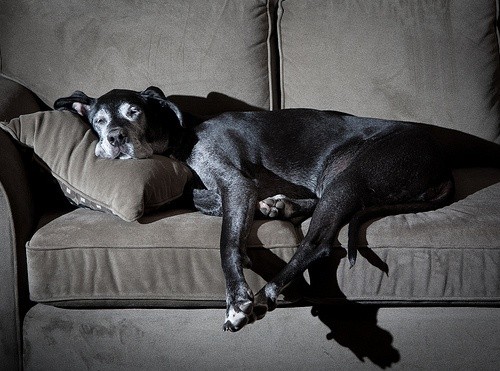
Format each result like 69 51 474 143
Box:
0 0 500 371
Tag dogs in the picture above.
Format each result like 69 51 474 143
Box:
53 87 457 332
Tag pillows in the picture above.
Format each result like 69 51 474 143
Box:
0 107 192 223
